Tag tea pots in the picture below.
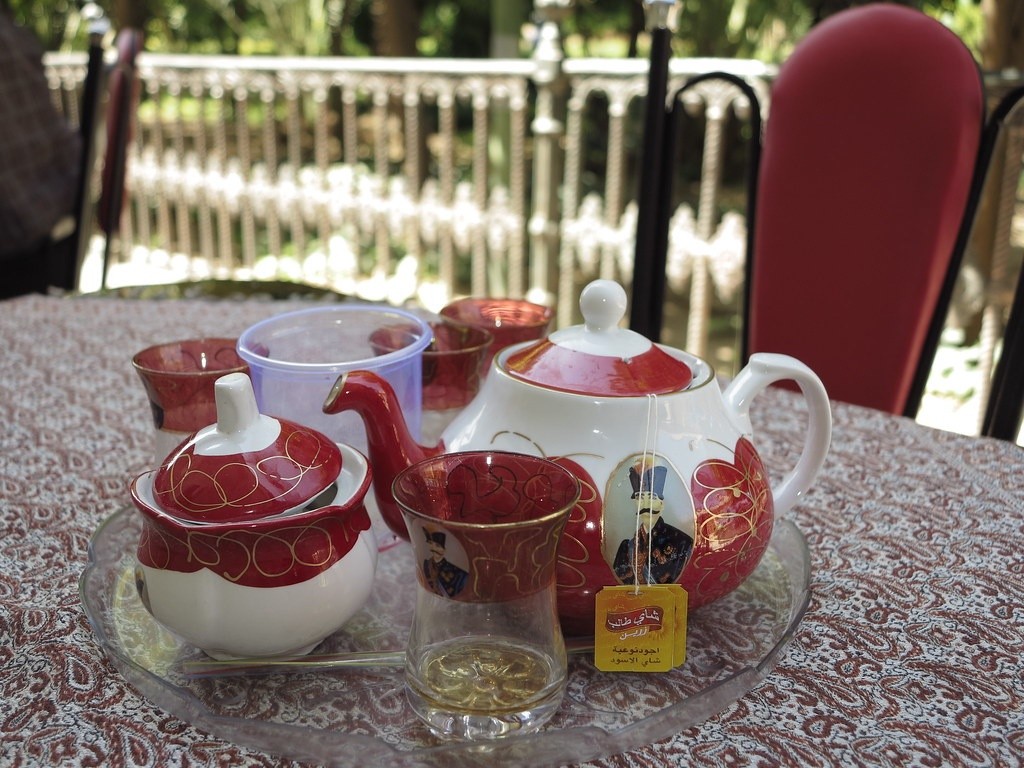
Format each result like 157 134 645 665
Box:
323 278 833 637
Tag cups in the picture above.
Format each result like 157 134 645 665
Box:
391 450 582 744
368 320 493 435
234 305 433 472
129 372 379 664
131 335 271 472
438 297 558 369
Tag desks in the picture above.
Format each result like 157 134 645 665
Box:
0 279 1024 768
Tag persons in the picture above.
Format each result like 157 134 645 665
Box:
0 0 79 282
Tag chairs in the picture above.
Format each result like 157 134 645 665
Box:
629 3 1023 446
57 24 143 293
